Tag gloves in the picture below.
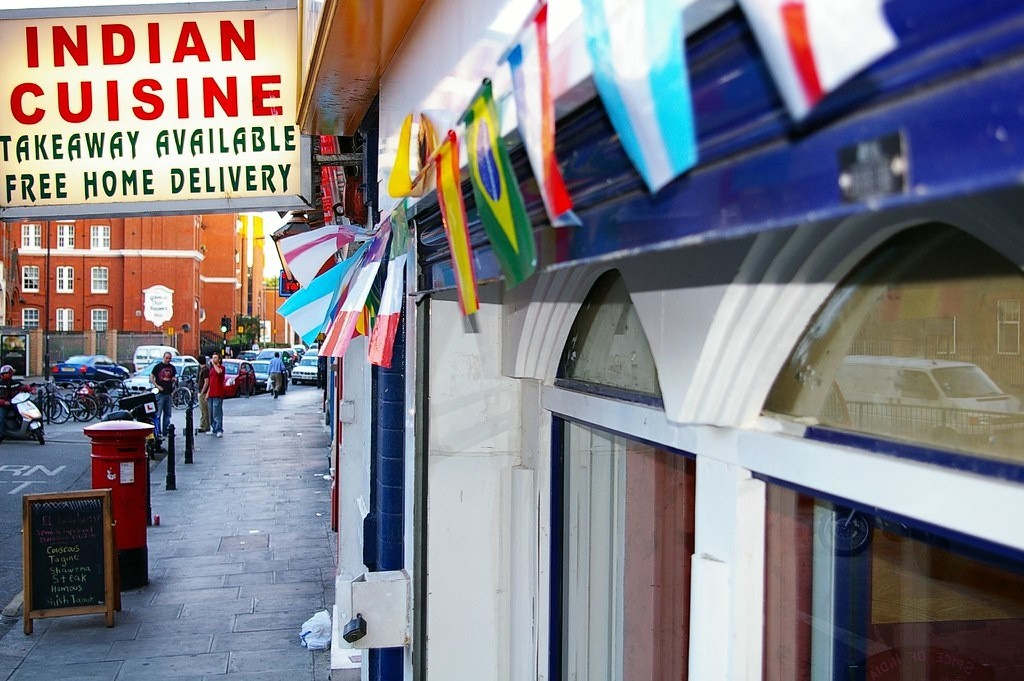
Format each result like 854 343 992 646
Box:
0 398 11 407
23 384 38 395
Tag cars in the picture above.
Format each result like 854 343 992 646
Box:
52 354 130 388
309 343 318 349
117 363 199 394
291 356 318 385
282 348 301 365
237 351 260 361
144 356 199 364
290 345 307 356
257 349 293 378
222 358 257 398
250 361 273 393
304 349 318 356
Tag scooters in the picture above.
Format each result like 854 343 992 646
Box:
815 506 906 557
0 392 45 445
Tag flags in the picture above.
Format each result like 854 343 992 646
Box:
276 0 902 369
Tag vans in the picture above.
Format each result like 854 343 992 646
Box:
838 354 1018 448
133 345 180 372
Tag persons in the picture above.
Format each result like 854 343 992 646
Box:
150 351 178 436
196 355 209 433
0 365 37 443
205 352 225 438
267 352 285 399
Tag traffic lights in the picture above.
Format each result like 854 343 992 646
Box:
221 317 227 333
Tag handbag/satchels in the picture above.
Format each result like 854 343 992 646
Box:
267 378 272 390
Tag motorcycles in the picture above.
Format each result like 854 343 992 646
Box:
119 388 165 458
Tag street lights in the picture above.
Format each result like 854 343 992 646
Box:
240 236 265 348
45 220 76 382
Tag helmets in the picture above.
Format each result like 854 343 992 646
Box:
0 365 16 380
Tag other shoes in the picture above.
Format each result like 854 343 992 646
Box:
206 431 217 436
198 428 211 433
217 432 223 438
159 437 165 441
271 389 279 399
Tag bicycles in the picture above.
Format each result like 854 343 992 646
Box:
31 374 199 424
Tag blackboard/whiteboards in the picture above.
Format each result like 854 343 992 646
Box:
22 487 113 618
